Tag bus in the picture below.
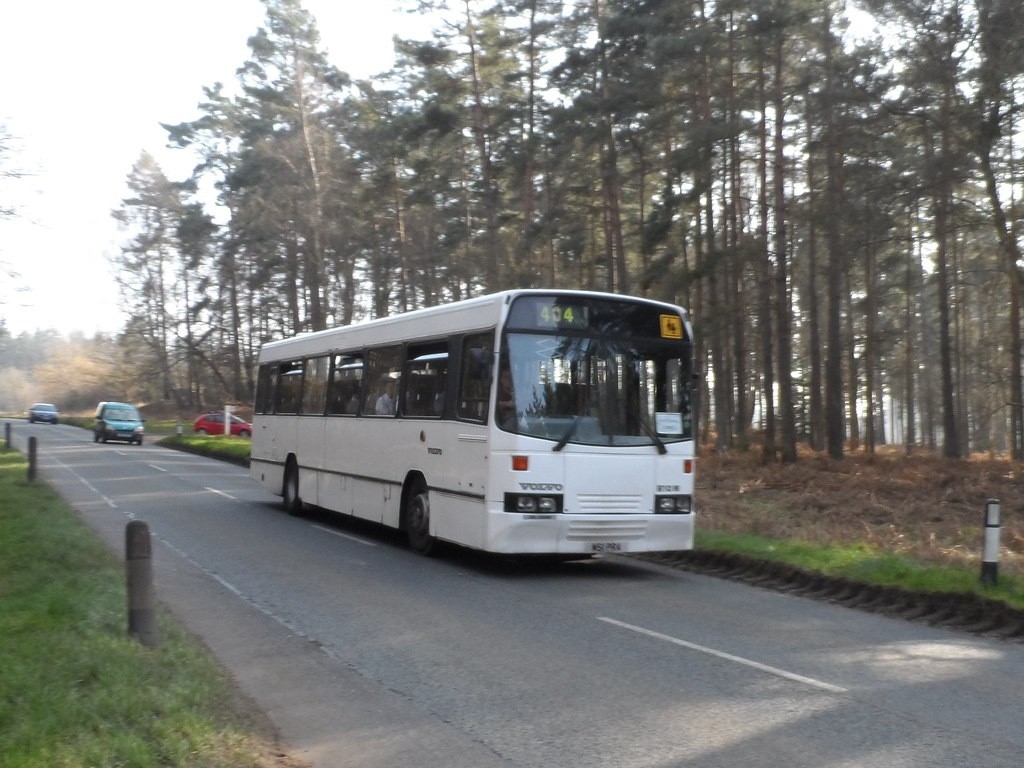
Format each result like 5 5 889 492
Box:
249 288 707 569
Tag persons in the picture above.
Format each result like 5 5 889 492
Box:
341 363 515 419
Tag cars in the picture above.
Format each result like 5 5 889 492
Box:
93 402 148 445
194 412 252 438
28 403 60 424
